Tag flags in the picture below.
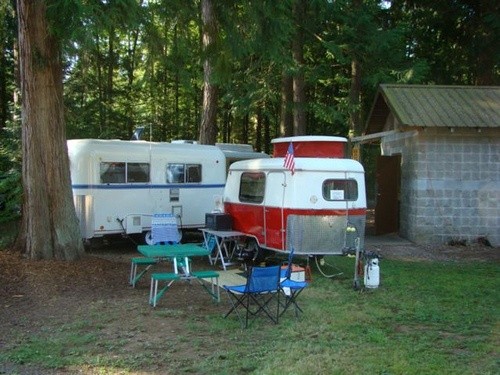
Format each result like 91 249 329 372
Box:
283 139 296 176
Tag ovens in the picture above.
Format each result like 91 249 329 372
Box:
205 212 232 231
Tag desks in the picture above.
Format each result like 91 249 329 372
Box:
130 242 221 307
198 227 245 271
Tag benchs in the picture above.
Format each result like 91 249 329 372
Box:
148 270 221 307
130 255 192 288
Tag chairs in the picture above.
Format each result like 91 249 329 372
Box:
224 265 280 330
145 214 182 244
255 248 296 318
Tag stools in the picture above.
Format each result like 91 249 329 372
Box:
278 277 307 318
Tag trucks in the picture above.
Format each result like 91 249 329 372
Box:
66 135 370 268
